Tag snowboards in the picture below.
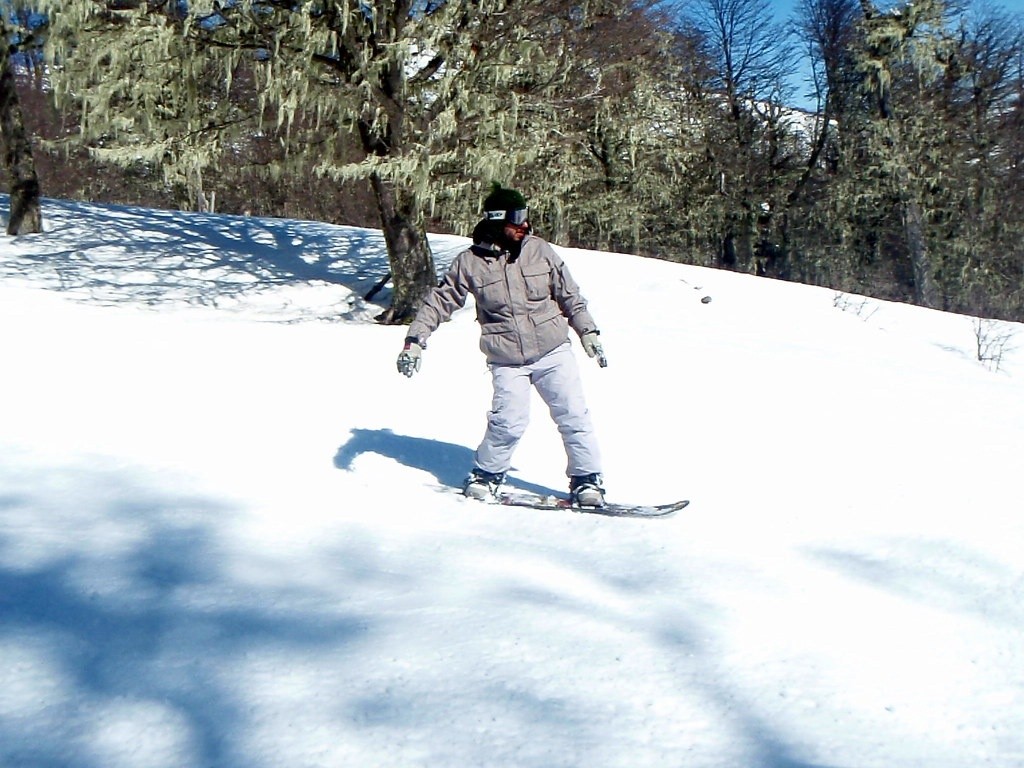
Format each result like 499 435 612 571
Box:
425 477 689 518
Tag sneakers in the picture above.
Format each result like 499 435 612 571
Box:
569 472 604 507
463 465 507 500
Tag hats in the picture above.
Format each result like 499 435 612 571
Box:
482 185 526 225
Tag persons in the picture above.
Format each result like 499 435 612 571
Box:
397 188 608 506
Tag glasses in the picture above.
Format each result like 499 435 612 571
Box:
506 206 531 225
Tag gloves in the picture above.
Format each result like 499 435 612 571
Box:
581 332 607 367
396 341 423 378
